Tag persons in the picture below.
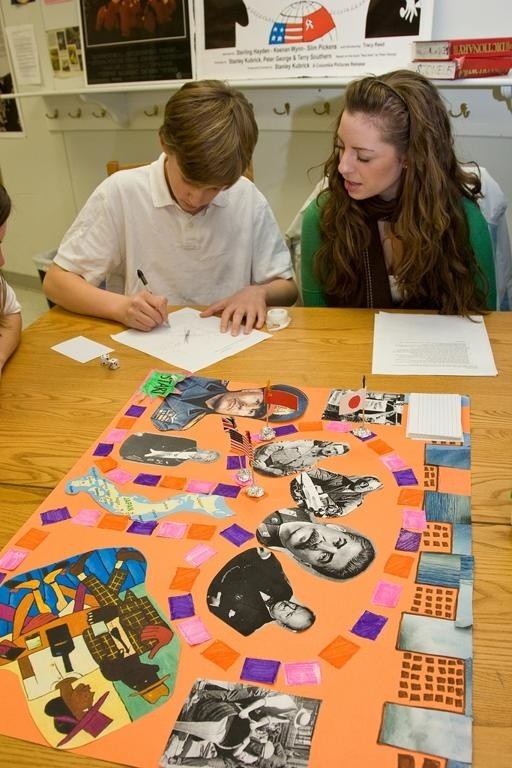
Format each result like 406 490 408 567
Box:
257 507 375 579
151 377 299 432
0 183 22 378
42 80 299 337
168 681 311 768
301 70 497 323
250 439 350 476
119 432 220 467
207 547 316 637
291 467 384 519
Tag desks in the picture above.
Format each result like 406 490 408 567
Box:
0 301 512 768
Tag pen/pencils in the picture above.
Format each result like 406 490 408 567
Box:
137 269 171 328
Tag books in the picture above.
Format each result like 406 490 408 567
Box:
409 57 512 80
411 37 512 61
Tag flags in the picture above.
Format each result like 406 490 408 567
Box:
222 416 237 433
338 388 367 415
230 429 254 460
266 380 273 402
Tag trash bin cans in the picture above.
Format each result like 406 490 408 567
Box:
34 246 106 309
